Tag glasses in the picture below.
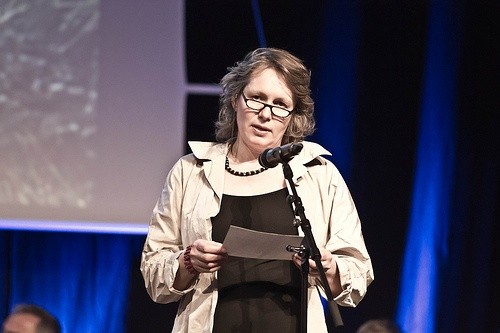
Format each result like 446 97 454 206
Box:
240 90 296 118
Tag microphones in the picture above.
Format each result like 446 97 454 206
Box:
258 141 303 170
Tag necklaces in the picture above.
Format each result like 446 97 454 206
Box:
225 149 271 176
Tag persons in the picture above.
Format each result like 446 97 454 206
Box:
1 304 63 333
354 319 404 333
139 47 375 333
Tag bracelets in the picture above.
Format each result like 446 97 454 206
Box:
183 245 199 276
